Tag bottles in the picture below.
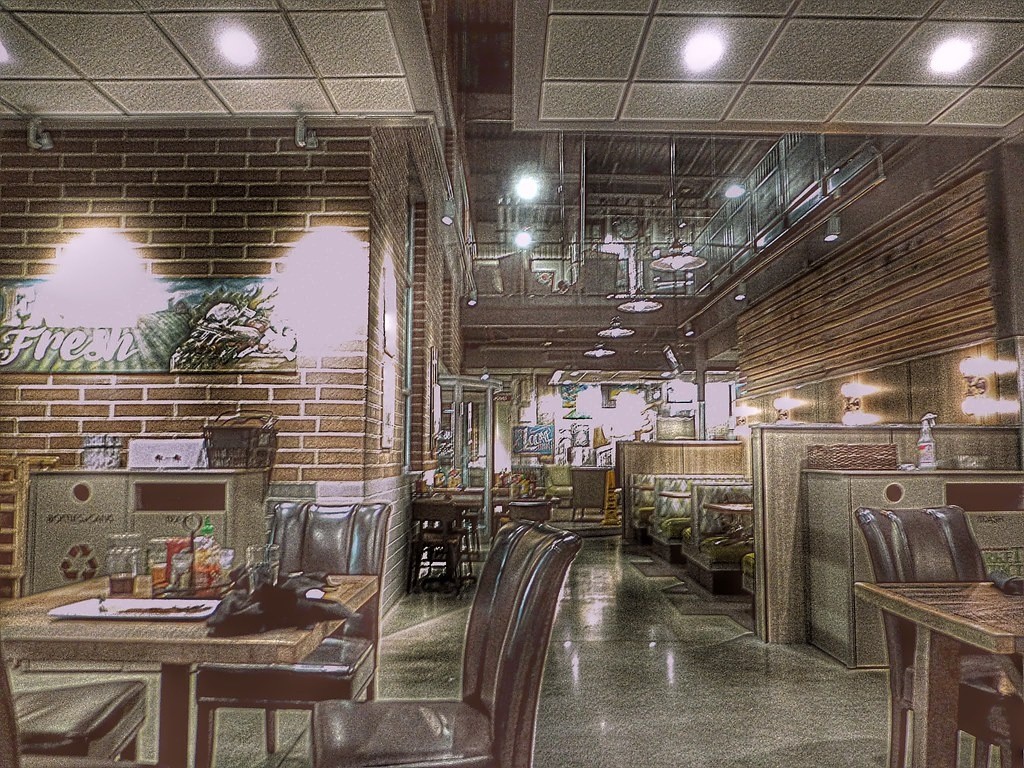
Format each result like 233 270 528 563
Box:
411 467 462 500
499 468 535 499
107 516 232 598
937 455 992 469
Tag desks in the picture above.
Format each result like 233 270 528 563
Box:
400 496 483 583
659 490 690 500
852 582 1024 768
702 504 755 517
0 574 379 768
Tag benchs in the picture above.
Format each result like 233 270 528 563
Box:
627 471 756 593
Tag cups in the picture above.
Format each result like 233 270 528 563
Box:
247 545 280 595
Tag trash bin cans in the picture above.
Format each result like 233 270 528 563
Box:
203 409 279 469
569 466 613 522
20 462 270 601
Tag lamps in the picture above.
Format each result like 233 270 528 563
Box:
597 314 634 339
824 214 841 241
25 117 55 150
584 343 613 359
733 279 749 302
294 116 320 151
616 231 663 312
684 321 694 338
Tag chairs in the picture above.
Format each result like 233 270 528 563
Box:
190 493 584 766
0 657 148 767
568 465 612 523
854 504 1017 768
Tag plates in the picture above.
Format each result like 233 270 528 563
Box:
46 597 222 619
